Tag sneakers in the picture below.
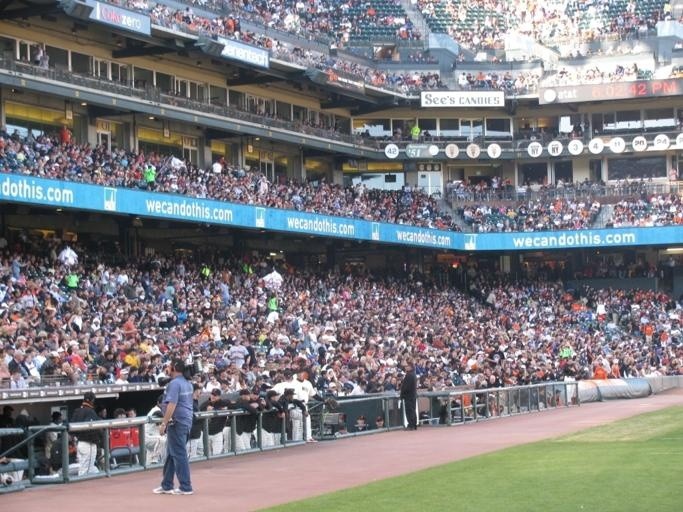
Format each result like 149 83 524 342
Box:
153 486 194 494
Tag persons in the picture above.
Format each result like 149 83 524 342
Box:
1 0 682 94
0 224 683 483
0 41 683 230
150 357 196 495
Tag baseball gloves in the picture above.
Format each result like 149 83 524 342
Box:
325 398 338 411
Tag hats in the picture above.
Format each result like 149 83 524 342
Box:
170 358 185 372
84 392 96 402
240 385 297 397
193 383 202 391
113 407 126 418
210 388 221 396
